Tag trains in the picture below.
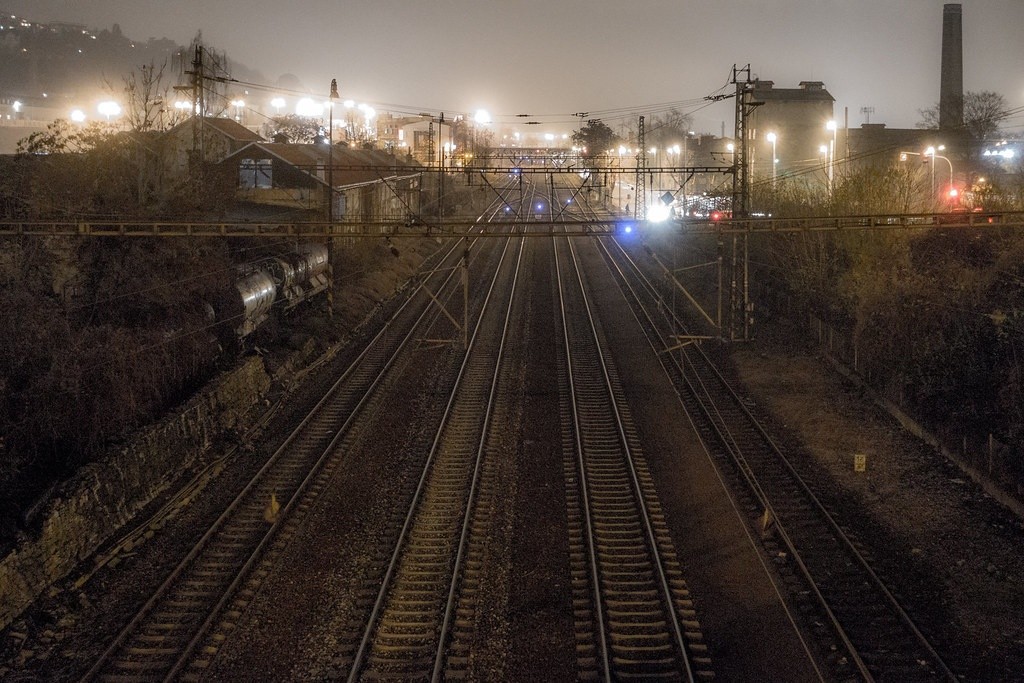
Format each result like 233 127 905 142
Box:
160 243 329 378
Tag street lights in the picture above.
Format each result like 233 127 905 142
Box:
728 144 734 181
99 102 118 134
326 79 339 318
763 130 779 190
438 112 445 233
900 151 953 188
922 145 941 199
820 118 837 188
821 145 827 196
618 145 626 210
683 132 694 199
932 144 946 204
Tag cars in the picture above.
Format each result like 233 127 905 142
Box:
717 210 773 228
932 208 993 223
